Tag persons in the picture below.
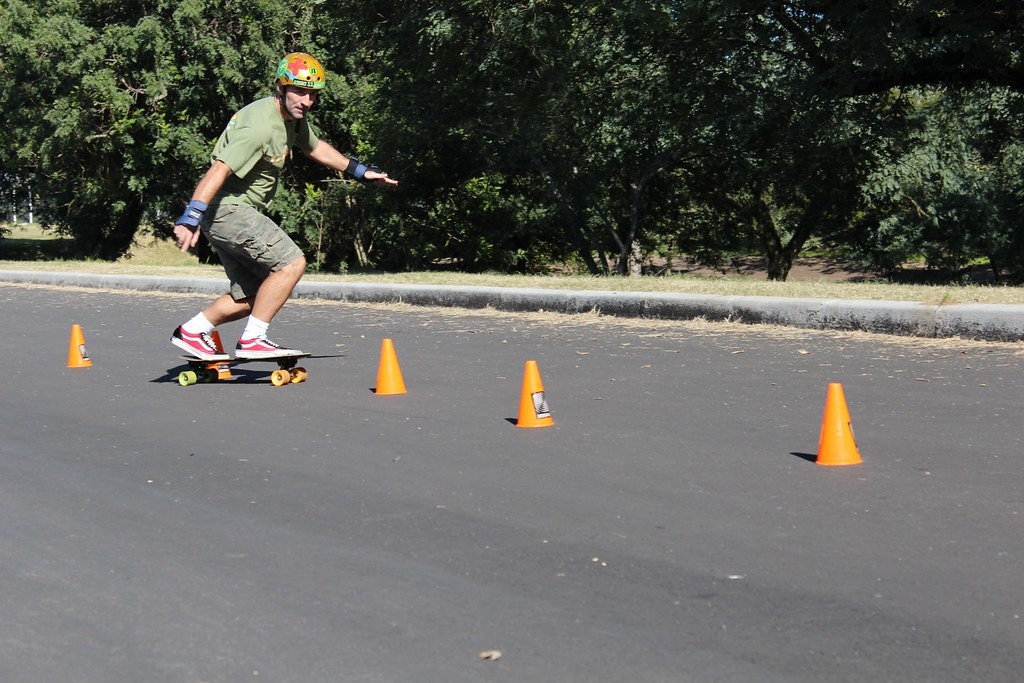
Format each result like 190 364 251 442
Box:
169 52 399 361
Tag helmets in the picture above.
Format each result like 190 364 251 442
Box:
274 52 325 90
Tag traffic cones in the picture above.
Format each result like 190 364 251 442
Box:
207 330 231 380
516 361 554 428
375 338 408 395
66 324 93 368
816 384 863 465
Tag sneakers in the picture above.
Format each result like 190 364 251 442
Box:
235 335 303 359
170 325 230 360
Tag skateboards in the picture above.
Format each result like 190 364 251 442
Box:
179 355 344 386
